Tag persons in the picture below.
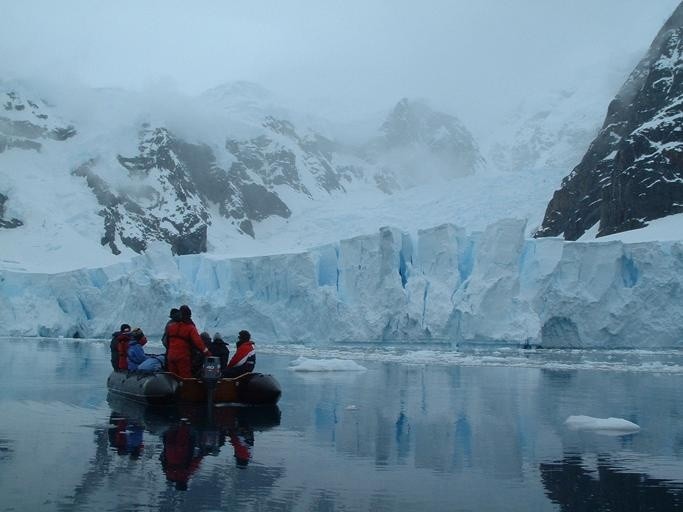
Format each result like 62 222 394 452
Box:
108 412 254 491
110 305 256 378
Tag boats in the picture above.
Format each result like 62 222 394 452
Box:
105 390 284 442
101 367 287 408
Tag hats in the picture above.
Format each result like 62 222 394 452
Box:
121 324 131 332
238 330 250 342
179 305 191 318
167 309 180 319
200 332 208 338
212 333 221 340
